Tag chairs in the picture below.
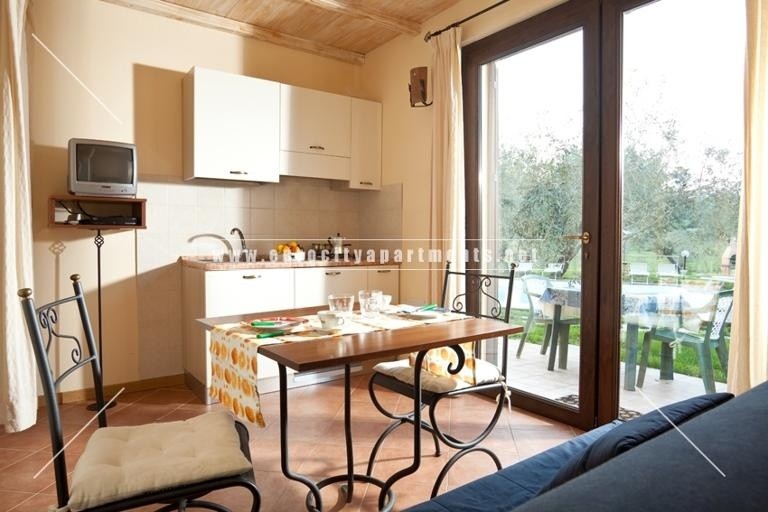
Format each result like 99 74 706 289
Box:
635 289 734 395
516 274 580 371
16 274 260 512
366 261 518 501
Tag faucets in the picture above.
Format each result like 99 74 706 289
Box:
230 228 248 259
187 233 233 258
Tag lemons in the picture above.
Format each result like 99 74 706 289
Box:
277 240 296 254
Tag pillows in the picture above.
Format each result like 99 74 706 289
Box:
529 392 735 498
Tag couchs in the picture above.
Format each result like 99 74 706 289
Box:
397 379 768 512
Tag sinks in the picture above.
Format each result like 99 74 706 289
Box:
194 258 261 263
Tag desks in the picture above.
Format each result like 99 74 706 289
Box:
536 284 733 391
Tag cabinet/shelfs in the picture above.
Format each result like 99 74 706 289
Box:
279 82 350 181
330 96 383 192
47 193 147 411
292 265 367 388
366 264 400 309
180 265 294 405
181 65 280 185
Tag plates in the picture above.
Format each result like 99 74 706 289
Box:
239 316 306 330
416 307 451 315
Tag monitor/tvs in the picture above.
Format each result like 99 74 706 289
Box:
67 138 138 199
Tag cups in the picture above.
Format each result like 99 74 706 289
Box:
318 289 393 328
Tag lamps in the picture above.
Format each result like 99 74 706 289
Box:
408 67 432 106
